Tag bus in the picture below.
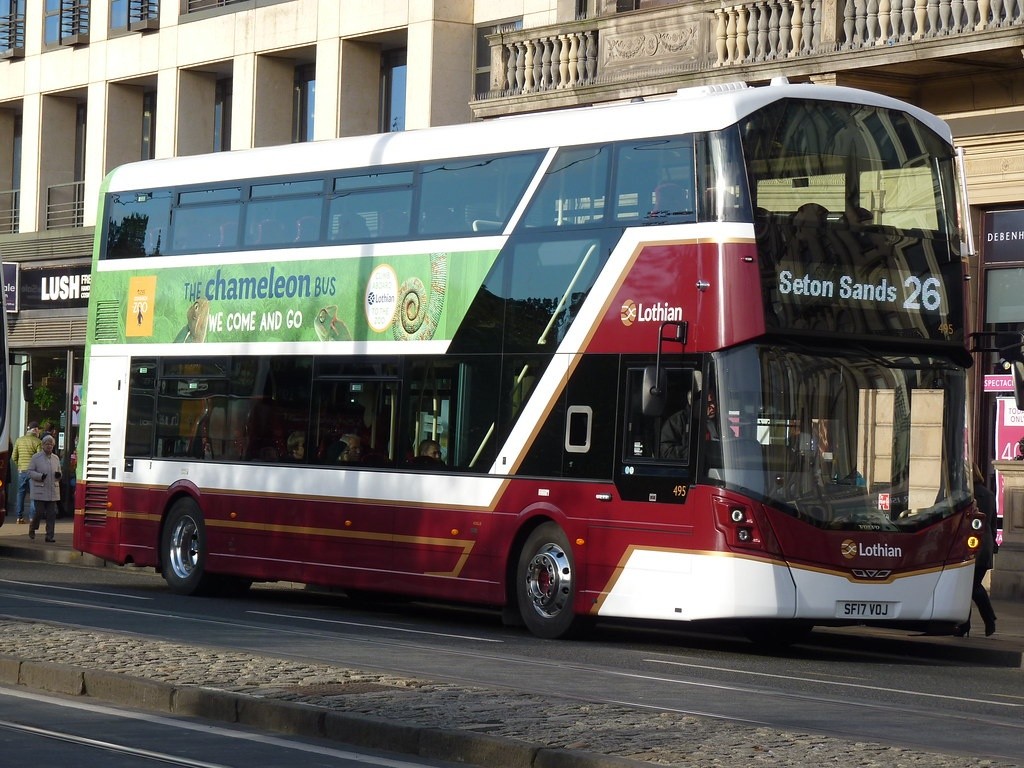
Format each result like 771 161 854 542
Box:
73 77 1024 639
0 255 34 527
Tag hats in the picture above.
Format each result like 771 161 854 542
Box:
325 441 348 466
27 421 39 428
843 207 873 224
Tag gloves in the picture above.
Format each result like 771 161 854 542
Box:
55 471 61 479
42 473 48 480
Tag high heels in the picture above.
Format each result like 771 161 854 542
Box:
957 619 971 637
985 616 997 636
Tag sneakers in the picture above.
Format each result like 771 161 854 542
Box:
16 518 26 524
45 537 55 543
29 524 35 539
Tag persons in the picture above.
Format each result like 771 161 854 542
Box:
41 422 56 441
338 434 362 461
288 431 305 458
419 440 440 460
954 508 999 636
12 421 43 524
28 435 63 542
661 389 720 459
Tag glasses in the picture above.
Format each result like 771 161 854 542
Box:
344 449 350 454
